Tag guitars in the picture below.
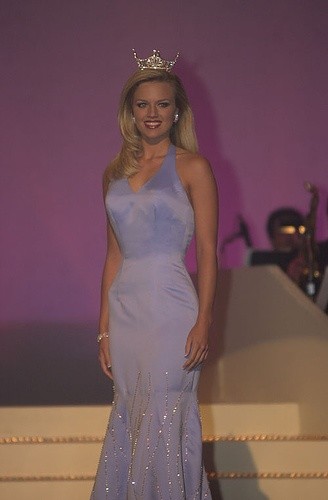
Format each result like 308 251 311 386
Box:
283 180 328 313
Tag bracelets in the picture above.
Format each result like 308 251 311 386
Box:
95 332 108 343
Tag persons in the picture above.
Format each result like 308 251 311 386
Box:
89 50 214 499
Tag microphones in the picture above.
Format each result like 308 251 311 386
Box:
238 215 253 249
